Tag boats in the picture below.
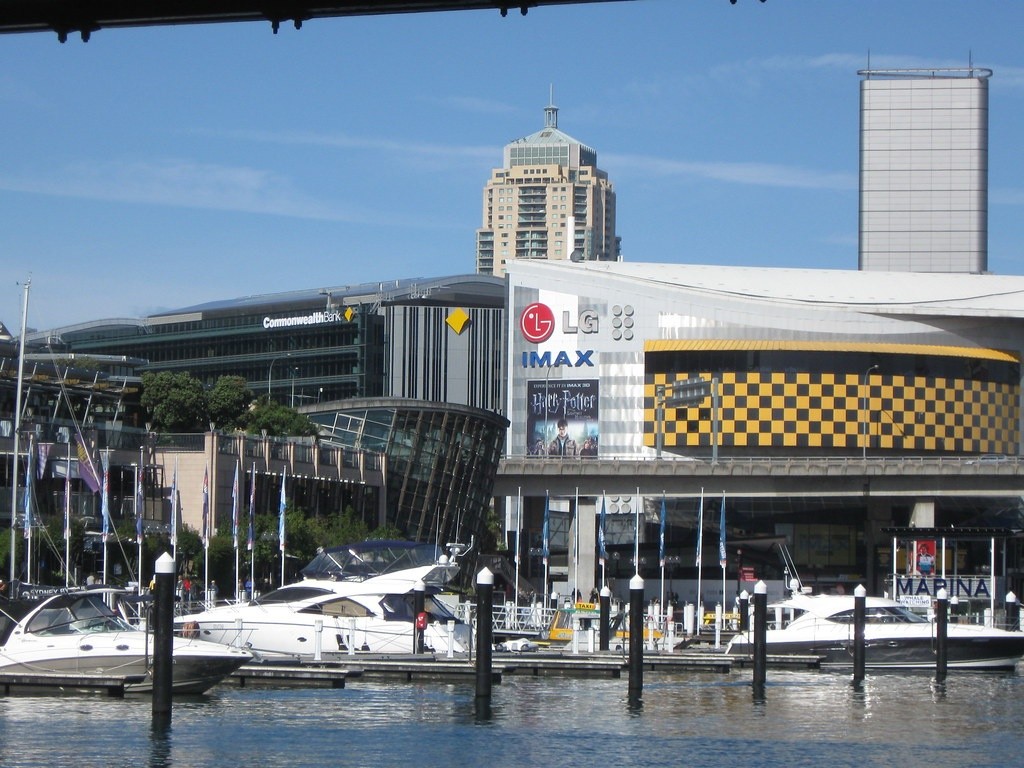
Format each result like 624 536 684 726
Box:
724 544 1024 668
0 586 259 694
174 506 476 655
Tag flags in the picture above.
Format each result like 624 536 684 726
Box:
719 495 727 568
695 497 702 567
542 495 550 565
573 497 579 564
170 473 177 545
202 467 210 549
514 496 522 565
24 433 144 545
231 464 240 550
247 464 256 550
277 472 287 551
633 504 638 566
598 497 609 565
659 496 666 567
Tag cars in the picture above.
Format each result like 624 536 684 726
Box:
539 603 662 642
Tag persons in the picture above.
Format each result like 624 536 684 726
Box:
917 543 935 575
209 580 218 593
579 440 594 456
548 419 577 454
245 577 251 599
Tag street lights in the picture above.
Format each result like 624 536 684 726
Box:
292 367 298 408
545 363 559 459
268 353 291 406
863 365 878 460
